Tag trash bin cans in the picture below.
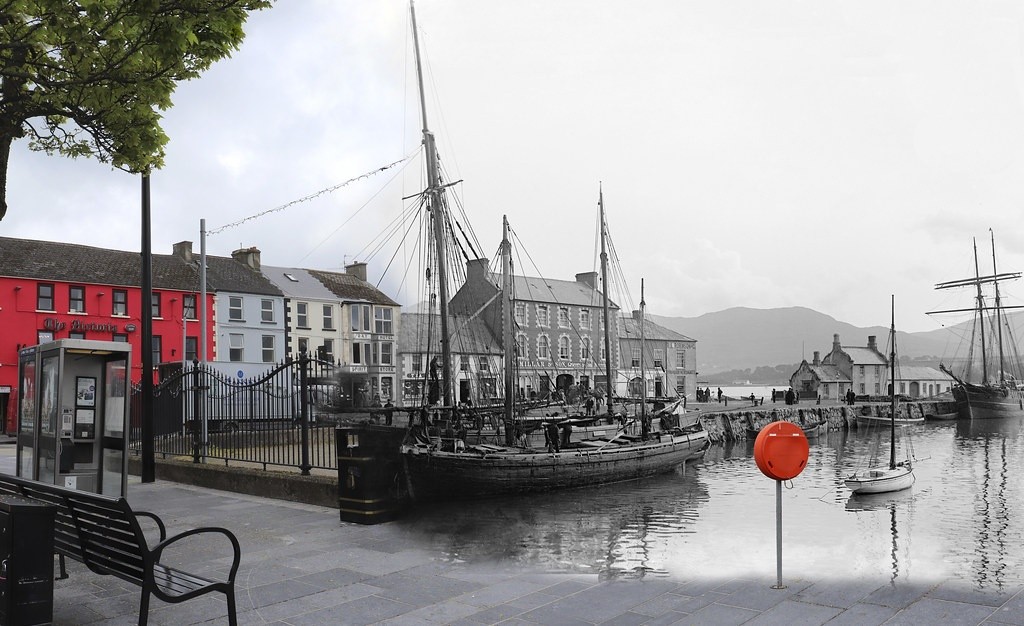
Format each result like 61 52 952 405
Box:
0 494 58 626
334 422 408 525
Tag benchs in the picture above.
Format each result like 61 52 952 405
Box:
0 472 241 626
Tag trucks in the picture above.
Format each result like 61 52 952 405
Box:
155 359 331 434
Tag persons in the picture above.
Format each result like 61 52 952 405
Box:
370 394 383 420
772 389 776 403
383 398 394 425
407 385 682 452
750 393 755 403
846 389 856 405
785 388 799 404
718 387 722 403
696 386 710 402
429 358 443 381
566 382 605 425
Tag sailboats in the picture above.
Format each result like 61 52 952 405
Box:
397 0 717 494
956 417 1024 593
845 295 917 494
924 228 1024 419
844 485 922 584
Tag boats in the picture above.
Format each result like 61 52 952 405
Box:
855 416 924 427
745 418 828 439
925 411 959 420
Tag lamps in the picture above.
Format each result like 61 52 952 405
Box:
171 298 177 302
125 323 136 332
16 285 22 292
98 292 104 296
